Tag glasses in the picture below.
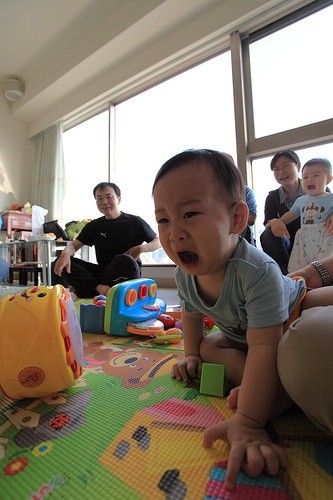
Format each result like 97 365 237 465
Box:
272 161 296 172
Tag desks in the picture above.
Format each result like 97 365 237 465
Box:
7 239 73 287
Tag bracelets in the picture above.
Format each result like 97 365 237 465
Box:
309 261 331 287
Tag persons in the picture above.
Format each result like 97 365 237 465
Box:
152 149 307 492
54 182 161 299
260 149 333 437
222 152 257 248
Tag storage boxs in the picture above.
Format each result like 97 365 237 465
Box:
1 212 32 230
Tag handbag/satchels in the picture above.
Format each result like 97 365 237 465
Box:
43 220 68 239
